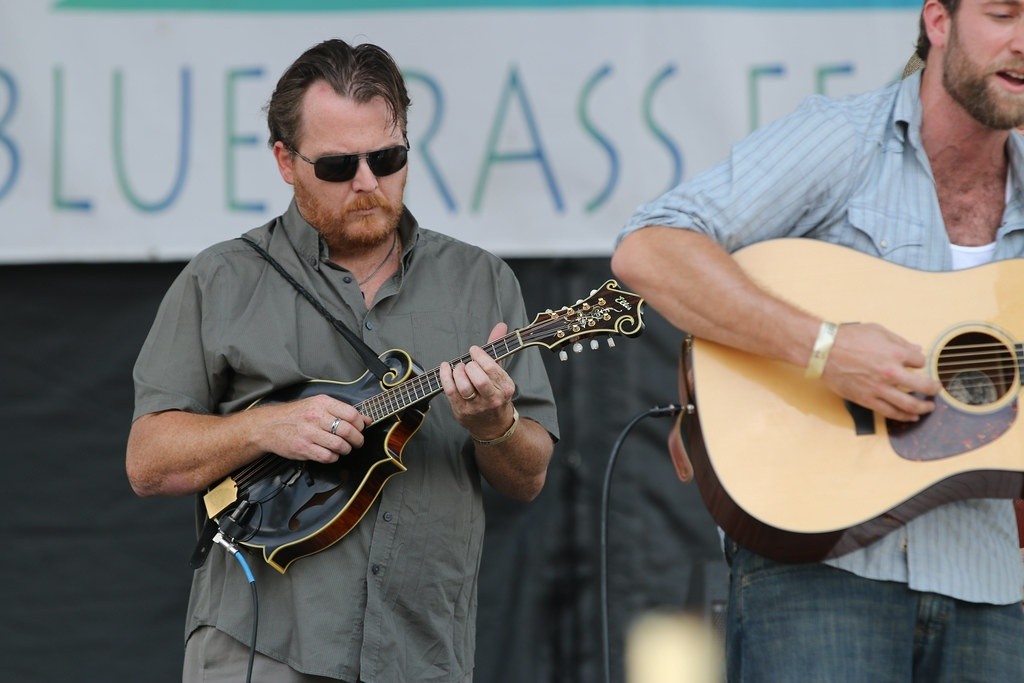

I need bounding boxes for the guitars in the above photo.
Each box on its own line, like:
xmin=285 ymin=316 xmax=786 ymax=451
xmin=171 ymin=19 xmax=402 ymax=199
xmin=677 ymin=234 xmax=1024 ymax=563
xmin=203 ymin=277 xmax=647 ymax=579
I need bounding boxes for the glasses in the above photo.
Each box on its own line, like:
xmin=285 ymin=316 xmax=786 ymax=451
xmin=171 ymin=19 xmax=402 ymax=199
xmin=285 ymin=134 xmax=411 ymax=182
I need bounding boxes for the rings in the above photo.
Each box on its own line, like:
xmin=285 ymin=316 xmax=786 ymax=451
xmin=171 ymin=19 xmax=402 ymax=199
xmin=461 ymin=393 xmax=477 ymax=401
xmin=330 ymin=415 xmax=341 ymax=435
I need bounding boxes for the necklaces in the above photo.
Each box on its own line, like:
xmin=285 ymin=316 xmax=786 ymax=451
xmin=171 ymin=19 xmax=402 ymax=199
xmin=358 ymin=232 xmax=397 ymax=300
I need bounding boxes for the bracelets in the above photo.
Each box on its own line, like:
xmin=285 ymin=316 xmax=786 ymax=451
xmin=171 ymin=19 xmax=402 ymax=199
xmin=801 ymin=318 xmax=837 ymax=386
xmin=468 ymin=408 xmax=519 ymax=446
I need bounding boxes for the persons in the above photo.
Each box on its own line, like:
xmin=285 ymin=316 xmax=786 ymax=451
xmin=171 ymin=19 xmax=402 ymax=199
xmin=611 ymin=2 xmax=1024 ymax=683
xmin=126 ymin=37 xmax=555 ymax=682
